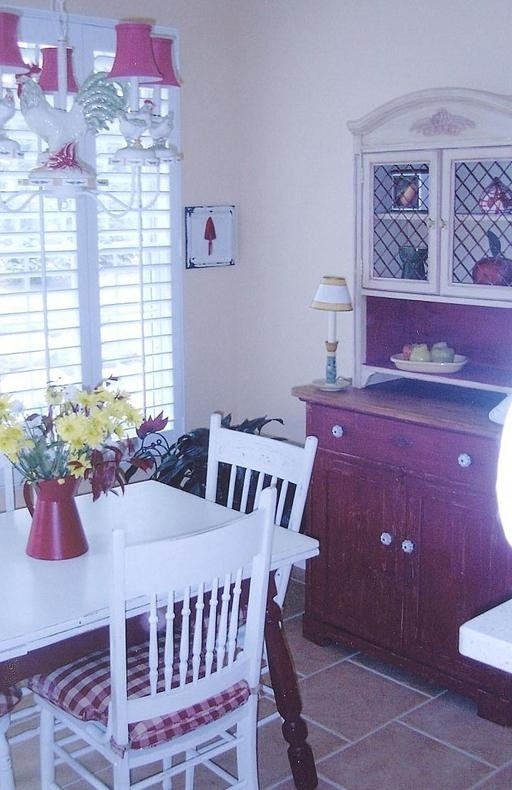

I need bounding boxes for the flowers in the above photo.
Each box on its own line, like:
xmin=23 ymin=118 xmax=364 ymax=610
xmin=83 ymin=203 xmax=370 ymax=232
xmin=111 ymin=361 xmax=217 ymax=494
xmin=0 ymin=375 xmax=169 ymax=502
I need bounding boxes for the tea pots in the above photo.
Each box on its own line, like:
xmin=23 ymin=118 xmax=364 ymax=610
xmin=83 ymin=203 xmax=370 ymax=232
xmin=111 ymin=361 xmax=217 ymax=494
xmin=22 ymin=475 xmax=91 ymax=561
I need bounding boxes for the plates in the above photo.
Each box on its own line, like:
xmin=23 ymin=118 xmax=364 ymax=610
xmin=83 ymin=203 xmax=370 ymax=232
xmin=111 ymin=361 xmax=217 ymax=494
xmin=390 ymin=352 xmax=469 ymax=375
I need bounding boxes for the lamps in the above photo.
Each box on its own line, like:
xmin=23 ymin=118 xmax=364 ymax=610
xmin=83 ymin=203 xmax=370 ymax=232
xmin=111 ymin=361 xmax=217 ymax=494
xmin=310 ymin=275 xmax=354 ymax=394
xmin=1 ymin=0 xmax=183 ymax=217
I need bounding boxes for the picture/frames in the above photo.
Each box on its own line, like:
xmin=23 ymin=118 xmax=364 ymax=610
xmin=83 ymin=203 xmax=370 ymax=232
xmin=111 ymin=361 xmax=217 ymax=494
xmin=184 ymin=204 xmax=237 ymax=270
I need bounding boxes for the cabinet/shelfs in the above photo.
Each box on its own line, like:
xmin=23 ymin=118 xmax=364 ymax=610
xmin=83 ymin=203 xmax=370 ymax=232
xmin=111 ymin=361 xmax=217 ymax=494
xmin=345 ymin=85 xmax=512 ymax=431
xmin=302 ymin=404 xmax=511 ymax=729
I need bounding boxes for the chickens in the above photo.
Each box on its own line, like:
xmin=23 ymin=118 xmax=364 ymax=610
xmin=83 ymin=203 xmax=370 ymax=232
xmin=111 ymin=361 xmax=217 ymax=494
xmin=130 ymin=110 xmax=174 ymax=149
xmin=0 ymin=90 xmax=18 ymax=135
xmin=11 ymin=60 xmax=128 ymax=173
xmin=115 ymin=101 xmax=155 ymax=148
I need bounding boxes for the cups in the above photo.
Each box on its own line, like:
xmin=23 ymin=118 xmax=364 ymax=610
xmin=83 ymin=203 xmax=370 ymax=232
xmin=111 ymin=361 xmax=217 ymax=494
xmin=398 ymin=244 xmax=428 ymax=280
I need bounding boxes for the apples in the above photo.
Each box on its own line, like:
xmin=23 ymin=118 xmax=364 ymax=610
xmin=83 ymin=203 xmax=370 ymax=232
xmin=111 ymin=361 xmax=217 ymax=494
xmin=403 ymin=339 xmax=456 ymax=365
xmin=472 ymin=257 xmax=512 ymax=285
